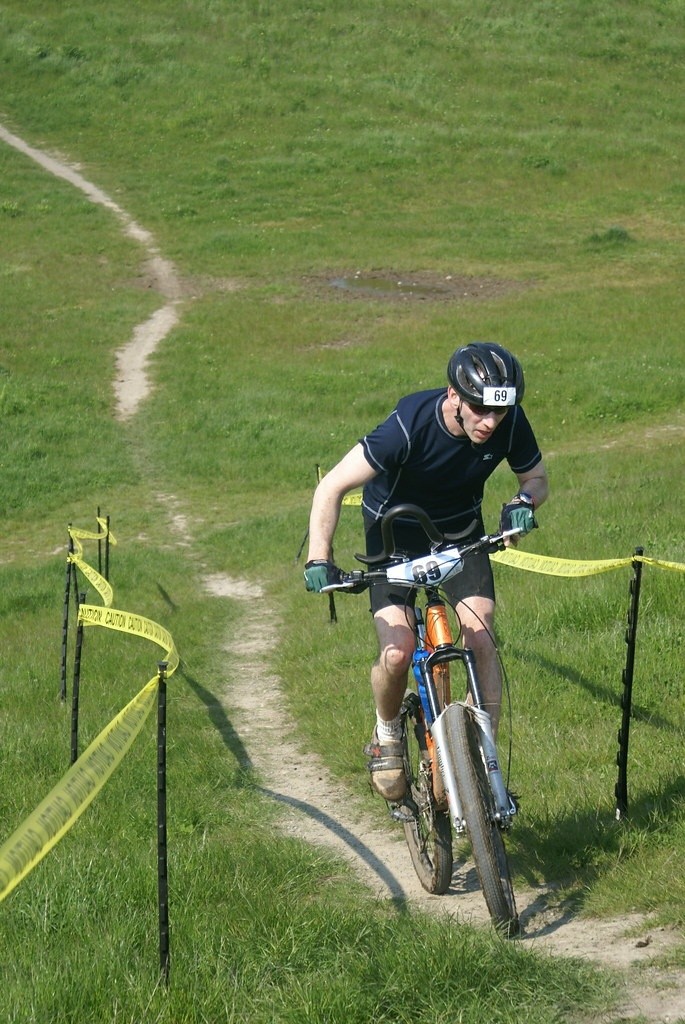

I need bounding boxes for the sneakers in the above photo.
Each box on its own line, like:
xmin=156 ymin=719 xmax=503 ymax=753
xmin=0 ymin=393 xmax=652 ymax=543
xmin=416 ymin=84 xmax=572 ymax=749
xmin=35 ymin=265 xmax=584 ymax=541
xmin=371 ymin=723 xmax=409 ymax=800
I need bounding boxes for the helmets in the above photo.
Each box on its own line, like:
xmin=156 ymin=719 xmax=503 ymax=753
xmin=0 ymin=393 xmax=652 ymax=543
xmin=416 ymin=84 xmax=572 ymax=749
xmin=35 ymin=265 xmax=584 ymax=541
xmin=446 ymin=341 xmax=525 ymax=405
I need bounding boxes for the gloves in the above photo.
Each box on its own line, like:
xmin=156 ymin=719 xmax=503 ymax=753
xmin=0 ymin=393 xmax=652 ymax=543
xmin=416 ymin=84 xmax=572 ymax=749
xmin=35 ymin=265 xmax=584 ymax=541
xmin=502 ymin=504 xmax=539 ymax=546
xmin=304 ymin=560 xmax=331 ymax=593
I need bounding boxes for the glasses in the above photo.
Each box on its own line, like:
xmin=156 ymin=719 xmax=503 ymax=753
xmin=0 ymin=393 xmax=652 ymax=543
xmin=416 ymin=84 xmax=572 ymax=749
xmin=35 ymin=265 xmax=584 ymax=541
xmin=468 ymin=404 xmax=508 ymax=415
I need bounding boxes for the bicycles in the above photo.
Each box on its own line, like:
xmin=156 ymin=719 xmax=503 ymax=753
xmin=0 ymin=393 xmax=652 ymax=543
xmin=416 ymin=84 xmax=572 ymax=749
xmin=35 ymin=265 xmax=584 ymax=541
xmin=318 ymin=527 xmax=521 ymax=942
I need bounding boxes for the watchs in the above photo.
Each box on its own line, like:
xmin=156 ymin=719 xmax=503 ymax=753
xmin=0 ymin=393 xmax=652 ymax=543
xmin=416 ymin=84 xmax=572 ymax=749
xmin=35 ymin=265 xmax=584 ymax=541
xmin=519 ymin=492 xmax=535 ymax=505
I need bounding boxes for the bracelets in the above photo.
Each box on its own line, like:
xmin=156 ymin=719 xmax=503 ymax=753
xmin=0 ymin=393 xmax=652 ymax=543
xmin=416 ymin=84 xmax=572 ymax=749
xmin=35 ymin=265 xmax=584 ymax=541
xmin=302 ymin=341 xmax=550 ymax=817
